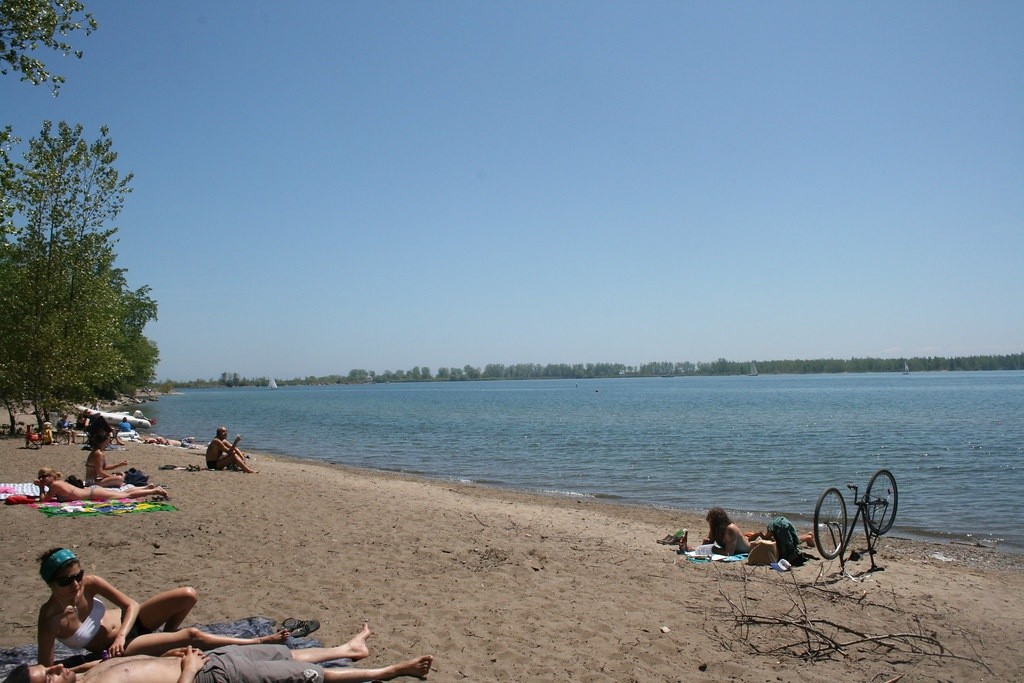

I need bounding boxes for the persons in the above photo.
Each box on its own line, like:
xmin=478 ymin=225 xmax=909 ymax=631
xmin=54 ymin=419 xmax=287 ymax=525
xmin=206 ymin=426 xmax=260 ymax=474
xmin=9 ymin=624 xmax=433 ymax=683
xmin=34 ymin=468 xmax=168 ymax=502
xmin=90 ymin=398 xmax=100 ymax=410
xmin=702 ymin=507 xmax=816 ymax=556
xmin=86 ymin=430 xmax=128 ymax=488
xmin=36 ymin=547 xmax=290 ymax=668
xmin=119 ymin=417 xmax=131 ymax=432
xmin=42 ymin=408 xmax=125 ymax=445
xmin=143 ymin=436 xmax=205 ymax=450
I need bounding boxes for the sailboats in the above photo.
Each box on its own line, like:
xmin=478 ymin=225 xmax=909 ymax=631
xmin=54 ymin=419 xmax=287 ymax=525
xmin=749 ymin=364 xmax=759 ymax=376
xmin=265 ymin=377 xmax=279 ymax=390
xmin=902 ymin=362 xmax=911 ymax=375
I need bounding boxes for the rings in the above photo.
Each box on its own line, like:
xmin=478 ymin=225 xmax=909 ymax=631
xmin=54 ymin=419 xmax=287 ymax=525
xmin=115 ymin=650 xmax=120 ymax=653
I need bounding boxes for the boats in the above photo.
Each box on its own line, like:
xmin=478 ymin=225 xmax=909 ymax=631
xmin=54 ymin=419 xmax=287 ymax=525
xmin=74 ymin=405 xmax=152 ymax=429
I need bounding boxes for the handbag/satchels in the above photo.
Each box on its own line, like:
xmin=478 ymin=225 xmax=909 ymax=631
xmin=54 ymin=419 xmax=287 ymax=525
xmin=679 ymin=530 xmax=695 ymax=552
xmin=124 ymin=468 xmax=149 ymax=487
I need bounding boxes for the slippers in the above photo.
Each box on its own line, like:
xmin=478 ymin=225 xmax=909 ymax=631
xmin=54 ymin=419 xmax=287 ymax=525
xmin=291 ymin=620 xmax=320 ymax=638
xmin=277 ymin=618 xmax=301 ymax=634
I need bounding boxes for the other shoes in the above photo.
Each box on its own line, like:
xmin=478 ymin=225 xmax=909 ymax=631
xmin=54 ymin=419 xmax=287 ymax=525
xmin=186 ymin=464 xmax=200 ymax=472
xmin=656 ymin=534 xmax=683 ymax=545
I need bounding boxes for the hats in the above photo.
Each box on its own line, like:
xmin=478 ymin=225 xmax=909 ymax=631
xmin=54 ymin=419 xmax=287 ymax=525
xmin=770 ymin=559 xmax=791 ymax=572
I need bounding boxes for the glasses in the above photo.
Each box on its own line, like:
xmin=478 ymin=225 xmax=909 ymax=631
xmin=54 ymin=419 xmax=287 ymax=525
xmin=38 ymin=475 xmax=51 ymax=479
xmin=50 ymin=569 xmax=84 ymax=587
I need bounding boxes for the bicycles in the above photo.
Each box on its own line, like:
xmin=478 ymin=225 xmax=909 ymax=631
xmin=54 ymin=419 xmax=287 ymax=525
xmin=810 ymin=469 xmax=899 ymax=586
